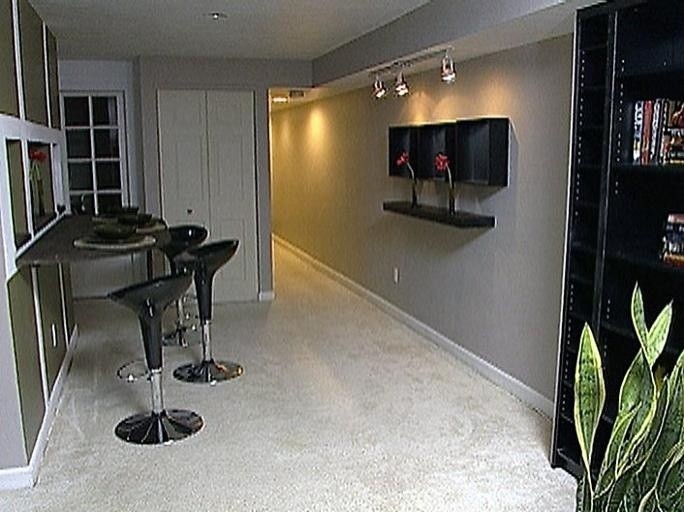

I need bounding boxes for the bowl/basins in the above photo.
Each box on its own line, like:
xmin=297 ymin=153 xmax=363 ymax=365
xmin=93 ymin=224 xmax=137 ymax=240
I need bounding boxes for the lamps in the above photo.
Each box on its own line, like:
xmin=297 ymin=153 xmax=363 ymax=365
xmin=371 ymin=51 xmax=455 ymax=100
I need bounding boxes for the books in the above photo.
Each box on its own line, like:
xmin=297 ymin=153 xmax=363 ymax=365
xmin=661 ymin=214 xmax=684 ymax=266
xmin=631 ymin=100 xmax=683 ymax=166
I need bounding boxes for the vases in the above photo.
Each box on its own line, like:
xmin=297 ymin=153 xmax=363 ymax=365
xmin=39 ymin=180 xmax=44 ymax=217
xmin=406 ymin=184 xmax=419 ymax=208
xmin=30 ymin=177 xmax=36 ymax=224
xmin=446 ymin=189 xmax=457 ymax=216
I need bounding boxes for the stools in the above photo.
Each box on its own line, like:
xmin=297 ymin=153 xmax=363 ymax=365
xmin=157 ymin=225 xmax=205 ymax=345
xmin=107 ymin=271 xmax=202 ymax=443
xmin=174 ymin=240 xmax=243 ymax=384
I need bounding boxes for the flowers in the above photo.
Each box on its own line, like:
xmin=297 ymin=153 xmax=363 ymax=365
xmin=434 ymin=154 xmax=456 ymax=189
xmin=396 ymin=152 xmax=414 ymax=183
xmin=30 ymin=147 xmax=45 ymax=181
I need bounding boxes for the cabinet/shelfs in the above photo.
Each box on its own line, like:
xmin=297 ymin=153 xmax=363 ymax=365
xmin=0 ymin=1 xmax=81 ymax=491
xmin=155 ymin=91 xmax=258 ymax=303
xmin=381 ymin=118 xmax=510 ymax=229
xmin=548 ymin=1 xmax=683 ymax=484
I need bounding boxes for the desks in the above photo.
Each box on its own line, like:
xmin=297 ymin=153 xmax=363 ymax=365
xmin=15 ymin=214 xmax=165 ymax=281
